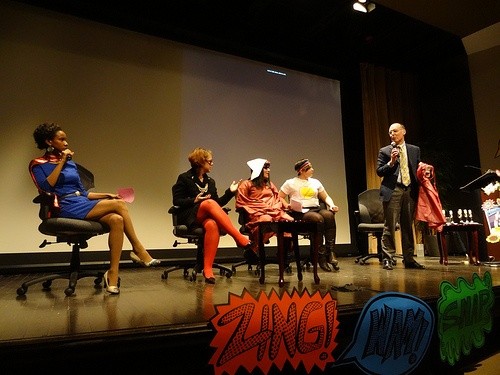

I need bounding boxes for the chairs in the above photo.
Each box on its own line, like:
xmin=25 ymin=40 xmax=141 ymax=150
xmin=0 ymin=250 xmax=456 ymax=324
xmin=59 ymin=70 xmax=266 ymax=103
xmin=231 ymin=206 xmax=293 ymax=278
xmin=288 ymin=195 xmax=340 ymax=272
xmin=354 ymin=188 xmax=403 ymax=266
xmin=161 ymin=183 xmax=234 ymax=282
xmin=16 ymin=162 xmax=121 ymax=298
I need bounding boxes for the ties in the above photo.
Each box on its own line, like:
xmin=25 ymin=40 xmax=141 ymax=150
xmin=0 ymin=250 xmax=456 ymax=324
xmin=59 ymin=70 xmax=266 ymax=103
xmin=398 ymin=145 xmax=411 ymax=187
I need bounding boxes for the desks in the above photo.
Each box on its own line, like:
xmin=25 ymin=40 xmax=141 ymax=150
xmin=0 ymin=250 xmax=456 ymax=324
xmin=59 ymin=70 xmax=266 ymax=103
xmin=436 ymin=224 xmax=483 ymax=266
xmin=257 ymin=219 xmax=325 ymax=288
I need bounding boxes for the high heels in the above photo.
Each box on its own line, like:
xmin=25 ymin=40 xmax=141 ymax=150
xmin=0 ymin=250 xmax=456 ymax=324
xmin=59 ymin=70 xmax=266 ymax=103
xmin=130 ymin=250 xmax=162 ymax=267
xmin=203 ymin=270 xmax=216 ymax=284
xmin=237 ymin=240 xmax=255 ymax=248
xmin=103 ymin=270 xmax=120 ymax=295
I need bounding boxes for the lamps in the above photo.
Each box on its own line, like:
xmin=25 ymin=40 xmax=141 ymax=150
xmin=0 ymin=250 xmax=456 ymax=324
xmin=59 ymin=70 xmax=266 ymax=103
xmin=352 ymin=0 xmax=376 ymax=13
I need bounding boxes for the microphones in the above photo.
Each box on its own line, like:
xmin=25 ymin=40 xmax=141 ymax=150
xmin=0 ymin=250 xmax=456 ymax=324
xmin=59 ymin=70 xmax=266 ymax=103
xmin=391 ymin=142 xmax=399 ymax=163
xmin=65 ymin=149 xmax=72 ymax=161
xmin=464 ymin=165 xmax=486 ymax=172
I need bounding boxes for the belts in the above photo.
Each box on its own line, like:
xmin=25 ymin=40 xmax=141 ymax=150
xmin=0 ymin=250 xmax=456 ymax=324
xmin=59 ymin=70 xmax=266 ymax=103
xmin=58 ymin=191 xmax=83 ymax=200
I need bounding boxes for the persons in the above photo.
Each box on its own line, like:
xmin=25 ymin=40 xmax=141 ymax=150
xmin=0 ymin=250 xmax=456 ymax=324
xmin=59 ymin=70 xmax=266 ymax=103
xmin=32 ymin=122 xmax=161 ymax=293
xmin=376 ymin=122 xmax=426 ymax=270
xmin=172 ymin=147 xmax=256 ymax=284
xmin=279 ymin=158 xmax=339 ymax=272
xmin=235 ymin=158 xmax=294 ymax=274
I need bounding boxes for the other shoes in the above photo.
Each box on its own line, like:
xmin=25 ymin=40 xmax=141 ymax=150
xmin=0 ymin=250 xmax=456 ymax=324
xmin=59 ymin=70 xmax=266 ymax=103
xmin=327 ymin=252 xmax=338 ymax=264
xmin=319 ymin=258 xmax=332 ymax=272
xmin=404 ymin=260 xmax=425 ymax=269
xmin=383 ymin=260 xmax=393 ymax=270
